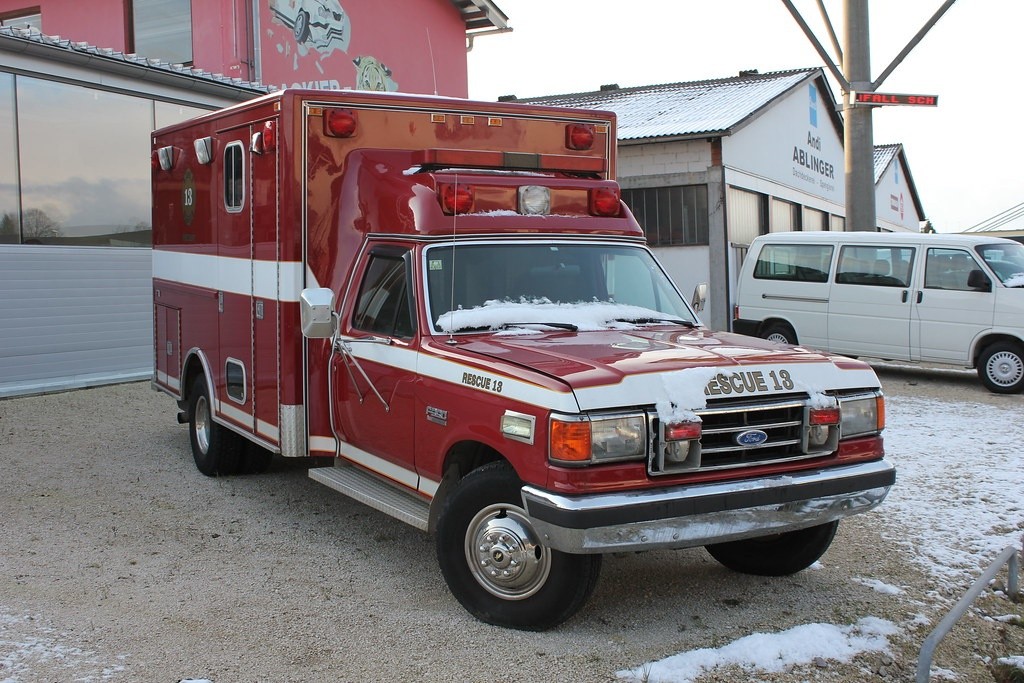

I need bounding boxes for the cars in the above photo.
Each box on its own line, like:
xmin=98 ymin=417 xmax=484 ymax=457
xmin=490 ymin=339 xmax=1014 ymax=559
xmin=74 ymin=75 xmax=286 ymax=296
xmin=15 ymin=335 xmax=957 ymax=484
xmin=268 ymin=0 xmax=347 ymax=53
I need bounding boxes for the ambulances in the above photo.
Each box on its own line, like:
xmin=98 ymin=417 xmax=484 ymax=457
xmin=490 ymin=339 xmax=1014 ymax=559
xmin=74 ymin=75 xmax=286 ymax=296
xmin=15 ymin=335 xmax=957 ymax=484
xmin=150 ymin=88 xmax=896 ymax=632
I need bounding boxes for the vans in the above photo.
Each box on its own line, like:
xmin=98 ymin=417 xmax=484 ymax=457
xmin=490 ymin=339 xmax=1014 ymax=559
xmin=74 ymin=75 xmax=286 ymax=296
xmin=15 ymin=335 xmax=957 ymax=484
xmin=733 ymin=231 xmax=1024 ymax=394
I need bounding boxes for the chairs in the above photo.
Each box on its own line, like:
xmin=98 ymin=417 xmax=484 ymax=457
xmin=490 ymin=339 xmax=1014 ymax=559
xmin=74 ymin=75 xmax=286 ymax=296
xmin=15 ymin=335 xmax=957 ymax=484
xmin=794 ymin=253 xmax=977 ymax=291
xmin=543 ymin=265 xmax=586 ymax=306
